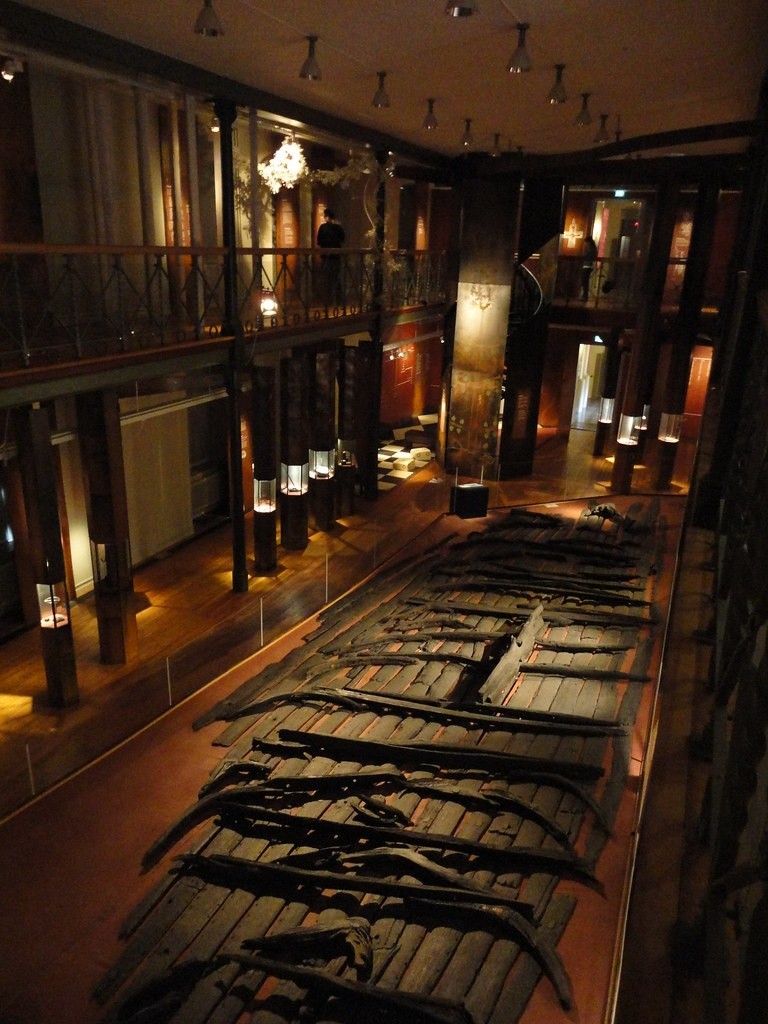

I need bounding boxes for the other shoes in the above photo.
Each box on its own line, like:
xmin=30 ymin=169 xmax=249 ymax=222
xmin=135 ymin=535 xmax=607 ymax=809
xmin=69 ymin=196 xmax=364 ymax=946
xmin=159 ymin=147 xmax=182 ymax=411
xmin=580 ymin=297 xmax=588 ymax=300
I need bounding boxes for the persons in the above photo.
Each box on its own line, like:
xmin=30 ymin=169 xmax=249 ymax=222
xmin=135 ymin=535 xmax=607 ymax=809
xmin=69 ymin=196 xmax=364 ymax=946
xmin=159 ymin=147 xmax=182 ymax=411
xmin=315 ymin=209 xmax=346 ymax=313
xmin=576 ymin=234 xmax=598 ymax=302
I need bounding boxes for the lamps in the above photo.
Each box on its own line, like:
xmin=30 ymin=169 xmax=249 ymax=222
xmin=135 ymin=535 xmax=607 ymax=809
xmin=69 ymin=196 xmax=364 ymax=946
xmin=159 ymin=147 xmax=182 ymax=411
xmin=1 ymin=61 xmax=16 ymax=81
xmin=193 ymin=0 xmax=612 ymax=158
